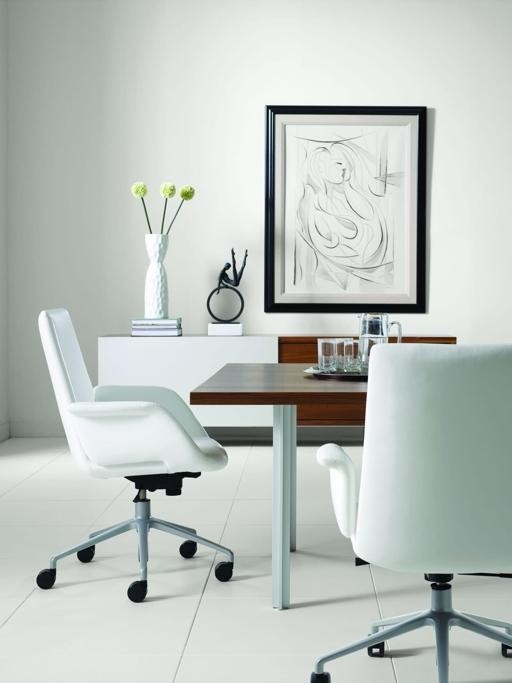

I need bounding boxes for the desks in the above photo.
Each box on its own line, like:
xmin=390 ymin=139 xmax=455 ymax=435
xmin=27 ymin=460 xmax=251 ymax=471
xmin=189 ymin=362 xmax=369 ymax=610
xmin=96 ymin=333 xmax=279 ymax=446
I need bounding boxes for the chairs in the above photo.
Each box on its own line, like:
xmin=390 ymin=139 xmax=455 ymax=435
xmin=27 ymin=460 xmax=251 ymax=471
xmin=310 ymin=342 xmax=512 ymax=683
xmin=36 ymin=305 xmax=234 ymax=602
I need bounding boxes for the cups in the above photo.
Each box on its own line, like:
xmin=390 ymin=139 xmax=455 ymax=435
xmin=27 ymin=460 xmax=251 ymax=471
xmin=318 ymin=335 xmax=354 ymax=372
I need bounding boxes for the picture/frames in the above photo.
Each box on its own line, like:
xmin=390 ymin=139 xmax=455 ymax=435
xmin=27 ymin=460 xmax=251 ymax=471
xmin=264 ymin=104 xmax=427 ymax=314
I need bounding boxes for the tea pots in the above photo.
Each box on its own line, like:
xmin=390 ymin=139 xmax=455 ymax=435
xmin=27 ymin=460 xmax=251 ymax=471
xmin=357 ymin=312 xmax=403 ymax=370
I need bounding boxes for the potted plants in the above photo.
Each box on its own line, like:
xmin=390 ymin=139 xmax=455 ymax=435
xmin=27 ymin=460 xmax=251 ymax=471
xmin=130 ymin=182 xmax=195 ymax=319
